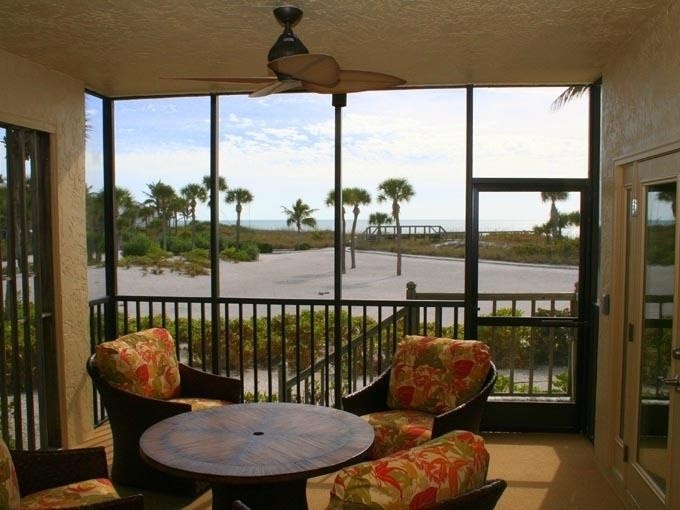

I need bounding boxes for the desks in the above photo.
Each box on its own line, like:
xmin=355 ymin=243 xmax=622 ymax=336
xmin=137 ymin=401 xmax=375 ymax=509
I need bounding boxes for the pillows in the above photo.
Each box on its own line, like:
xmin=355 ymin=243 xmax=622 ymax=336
xmin=94 ymin=327 xmax=181 ymax=401
xmin=0 ymin=438 xmax=21 ymax=509
xmin=325 ymin=429 xmax=491 ymax=510
xmin=385 ymin=333 xmax=491 ymax=413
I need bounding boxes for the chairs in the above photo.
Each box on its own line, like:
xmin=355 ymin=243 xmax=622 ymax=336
xmin=232 ymin=430 xmax=508 ymax=510
xmin=0 ymin=435 xmax=145 ymax=510
xmin=86 ymin=326 xmax=243 ymax=499
xmin=342 ymin=334 xmax=499 ymax=460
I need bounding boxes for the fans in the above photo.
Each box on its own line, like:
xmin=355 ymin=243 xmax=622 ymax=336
xmin=157 ymin=1 xmax=409 ymax=99
